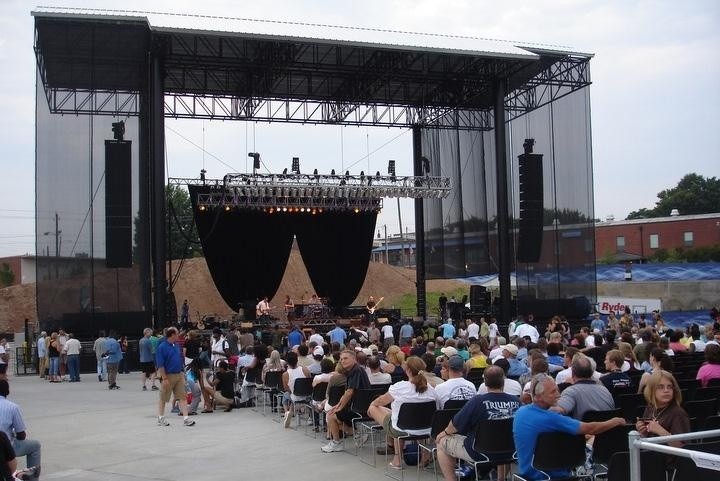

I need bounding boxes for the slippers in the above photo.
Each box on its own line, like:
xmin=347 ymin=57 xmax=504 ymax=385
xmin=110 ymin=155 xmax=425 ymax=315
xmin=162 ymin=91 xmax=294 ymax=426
xmin=387 ymin=460 xmax=403 ymax=470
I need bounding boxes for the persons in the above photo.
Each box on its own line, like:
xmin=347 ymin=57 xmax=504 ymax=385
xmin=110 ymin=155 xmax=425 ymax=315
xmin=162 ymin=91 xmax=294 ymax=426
xmin=254 ymin=296 xmax=720 ymax=480
xmin=0 ymin=336 xmax=10 ymax=380
xmin=38 ymin=327 xmax=255 ymax=427
xmin=0 ymin=380 xmax=41 ymax=481
xmin=0 ymin=431 xmax=18 ymax=481
xmin=181 ymin=300 xmax=189 ymax=323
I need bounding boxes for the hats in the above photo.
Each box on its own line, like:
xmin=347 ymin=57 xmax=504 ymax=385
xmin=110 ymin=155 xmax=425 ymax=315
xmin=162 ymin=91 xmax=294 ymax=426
xmin=440 ymin=345 xmax=458 ymax=358
xmin=312 ymin=346 xmax=325 ymax=358
xmin=500 ymin=343 xmax=519 ymax=356
xmin=447 ymin=355 xmax=465 ymax=370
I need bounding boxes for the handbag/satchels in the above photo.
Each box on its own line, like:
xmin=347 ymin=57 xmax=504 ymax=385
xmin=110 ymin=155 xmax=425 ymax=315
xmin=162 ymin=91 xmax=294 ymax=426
xmin=403 ymin=443 xmax=421 ymax=467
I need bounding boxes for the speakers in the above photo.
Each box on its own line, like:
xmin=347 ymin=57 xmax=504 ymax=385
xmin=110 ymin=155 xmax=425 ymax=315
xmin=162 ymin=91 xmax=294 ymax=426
xmin=104 ymin=140 xmax=132 ymax=268
xmin=479 ymin=292 xmax=491 ymax=312
xmin=470 ymin=285 xmax=486 ymax=313
xmin=518 ymin=154 xmax=543 ymax=263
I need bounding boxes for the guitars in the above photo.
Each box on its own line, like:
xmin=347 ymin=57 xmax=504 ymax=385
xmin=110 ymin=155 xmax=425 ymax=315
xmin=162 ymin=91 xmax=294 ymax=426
xmin=197 ymin=311 xmax=205 ymax=329
xmin=368 ymin=297 xmax=384 ymax=314
xmin=256 ymin=306 xmax=277 ymax=315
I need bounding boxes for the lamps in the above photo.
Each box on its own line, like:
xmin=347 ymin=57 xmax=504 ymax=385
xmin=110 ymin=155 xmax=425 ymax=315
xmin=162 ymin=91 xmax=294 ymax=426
xmin=195 ymin=183 xmax=452 ymax=214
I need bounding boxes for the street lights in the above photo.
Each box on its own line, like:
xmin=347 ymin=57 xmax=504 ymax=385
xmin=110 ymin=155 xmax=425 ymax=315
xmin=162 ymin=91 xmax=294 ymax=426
xmin=377 ymin=224 xmax=389 ymax=264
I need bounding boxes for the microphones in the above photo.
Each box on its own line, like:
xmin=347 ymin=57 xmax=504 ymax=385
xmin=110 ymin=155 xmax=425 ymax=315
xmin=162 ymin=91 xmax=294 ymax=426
xmin=257 ymin=298 xmax=258 ymax=300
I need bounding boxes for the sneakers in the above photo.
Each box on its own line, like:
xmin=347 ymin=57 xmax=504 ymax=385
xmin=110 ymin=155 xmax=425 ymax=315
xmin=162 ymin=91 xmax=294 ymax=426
xmin=282 ymin=411 xmax=292 ymax=428
xmin=376 ymin=444 xmax=394 ymax=455
xmin=321 ymin=439 xmax=345 ymax=453
xmin=152 ymin=385 xmax=160 ymax=392
xmin=98 ymin=374 xmax=106 ymax=381
xmin=141 ymin=385 xmax=146 ymax=391
xmin=108 ymin=383 xmax=120 ymax=390
xmin=200 ymin=408 xmax=213 ymax=413
xmin=40 ymin=375 xmax=80 ymax=383
xmin=171 ymin=405 xmax=179 ymax=413
xmin=157 ymin=416 xmax=170 ymax=427
xmin=454 ymin=462 xmax=475 ymax=478
xmin=223 ymin=404 xmax=235 ymax=412
xmin=417 ymin=451 xmax=435 ymax=469
xmin=307 ymin=415 xmax=357 ymax=440
xmin=353 ymin=432 xmax=369 ymax=448
xmin=183 ymin=417 xmax=196 ymax=425
